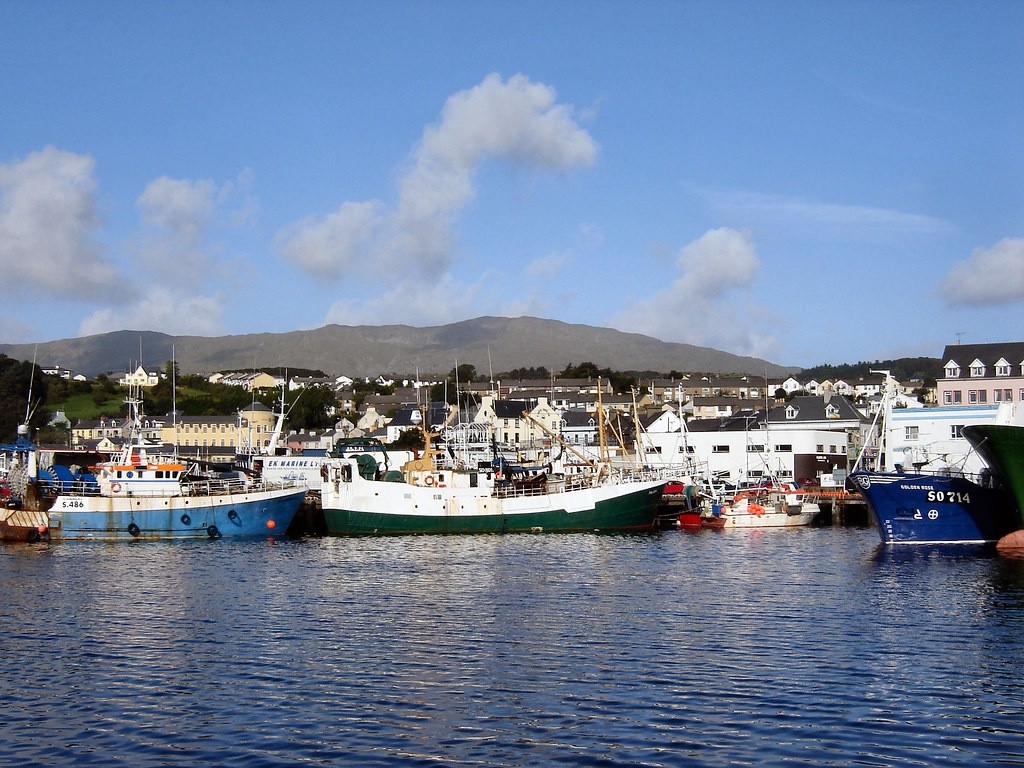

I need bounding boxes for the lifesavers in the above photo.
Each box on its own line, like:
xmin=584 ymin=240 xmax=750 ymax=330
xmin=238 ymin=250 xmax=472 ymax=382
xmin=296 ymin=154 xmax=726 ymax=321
xmin=228 ymin=510 xmax=237 ymax=519
xmin=207 ymin=525 xmax=218 ymax=537
xmin=110 ymin=481 xmax=121 ymax=493
xmin=181 ymin=514 xmax=190 ymax=523
xmin=127 ymin=523 xmax=139 ymax=534
xmin=425 ymin=475 xmax=435 ymax=485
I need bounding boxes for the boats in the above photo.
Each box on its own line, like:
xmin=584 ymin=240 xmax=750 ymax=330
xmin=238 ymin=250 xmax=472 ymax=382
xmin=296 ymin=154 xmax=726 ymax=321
xmin=842 ymin=369 xmax=1024 ymax=556
xmin=0 ymin=333 xmax=820 ymax=542
